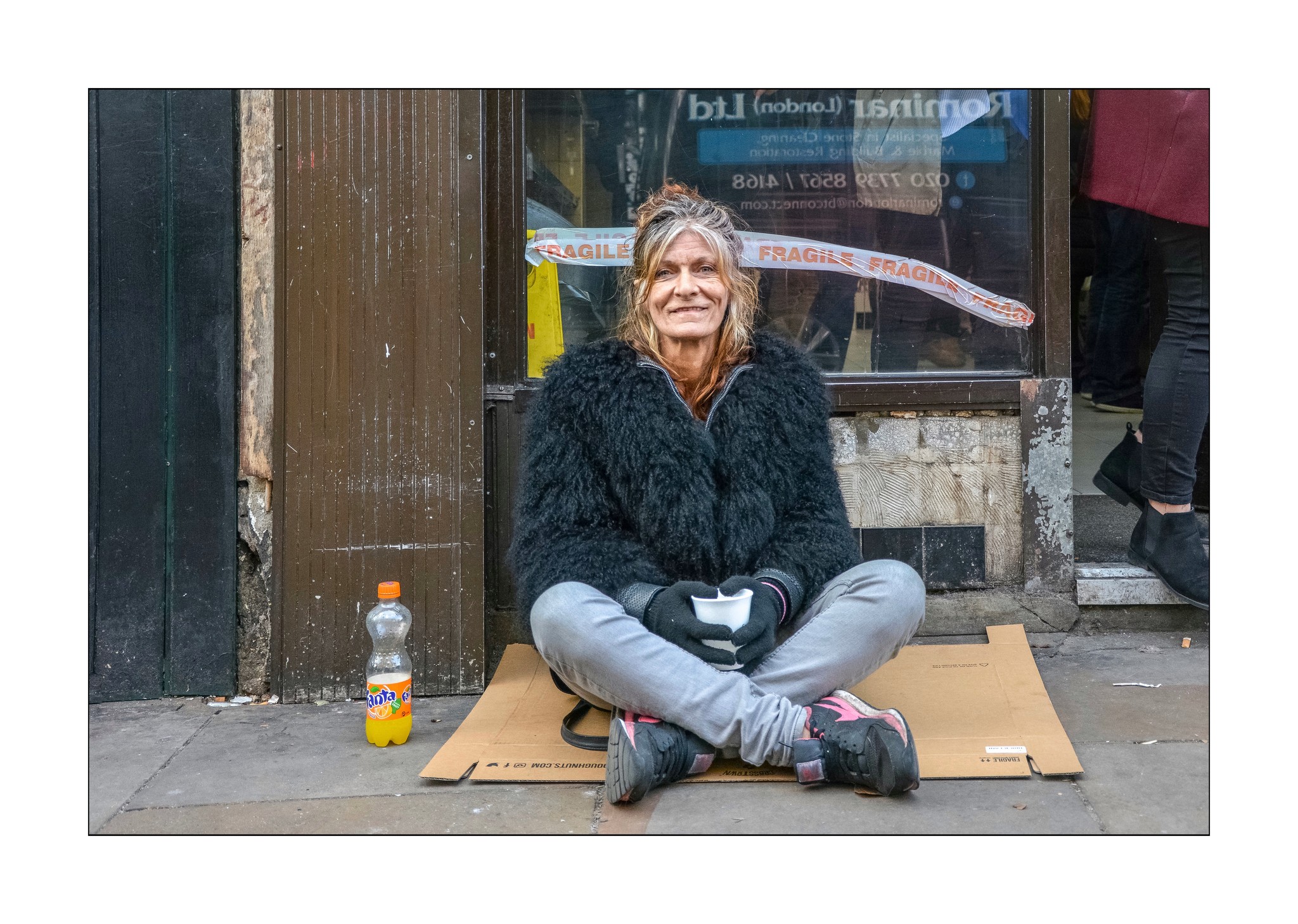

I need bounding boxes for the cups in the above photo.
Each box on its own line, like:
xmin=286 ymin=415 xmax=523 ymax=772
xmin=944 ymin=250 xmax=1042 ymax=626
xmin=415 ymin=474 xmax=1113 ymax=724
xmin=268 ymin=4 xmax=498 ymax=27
xmin=691 ymin=588 xmax=753 ymax=671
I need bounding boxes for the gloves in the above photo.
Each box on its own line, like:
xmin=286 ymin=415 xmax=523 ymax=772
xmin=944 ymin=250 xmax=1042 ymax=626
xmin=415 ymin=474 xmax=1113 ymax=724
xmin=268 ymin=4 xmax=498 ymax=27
xmin=645 ymin=580 xmax=736 ymax=666
xmin=720 ymin=576 xmax=777 ymax=676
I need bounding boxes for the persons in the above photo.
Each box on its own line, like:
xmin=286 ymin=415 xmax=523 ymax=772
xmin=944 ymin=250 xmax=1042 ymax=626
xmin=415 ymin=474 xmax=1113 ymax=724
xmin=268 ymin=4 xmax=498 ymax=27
xmin=1079 ymin=88 xmax=1210 ymax=608
xmin=854 ymin=88 xmax=1168 ymax=412
xmin=638 ymin=89 xmax=875 ymax=373
xmin=505 ymin=178 xmax=926 ymax=805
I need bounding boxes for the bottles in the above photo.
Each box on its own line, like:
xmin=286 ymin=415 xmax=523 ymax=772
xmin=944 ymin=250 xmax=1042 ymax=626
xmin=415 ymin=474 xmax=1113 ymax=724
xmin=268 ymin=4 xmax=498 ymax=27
xmin=366 ymin=581 xmax=413 ymax=747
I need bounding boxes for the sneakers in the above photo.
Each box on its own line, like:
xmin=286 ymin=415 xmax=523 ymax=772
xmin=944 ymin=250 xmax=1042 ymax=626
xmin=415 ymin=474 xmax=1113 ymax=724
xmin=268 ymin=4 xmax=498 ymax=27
xmin=606 ymin=703 xmax=719 ymax=805
xmin=792 ymin=689 xmax=922 ymax=797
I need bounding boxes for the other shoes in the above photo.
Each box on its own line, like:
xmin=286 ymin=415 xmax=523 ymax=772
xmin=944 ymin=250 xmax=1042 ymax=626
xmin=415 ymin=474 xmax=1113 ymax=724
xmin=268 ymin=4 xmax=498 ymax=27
xmin=1129 ymin=501 xmax=1210 ymax=614
xmin=1094 ymin=422 xmax=1210 ymax=513
xmin=1089 ymin=386 xmax=1146 ymax=413
xmin=1081 ymin=380 xmax=1093 ymax=399
